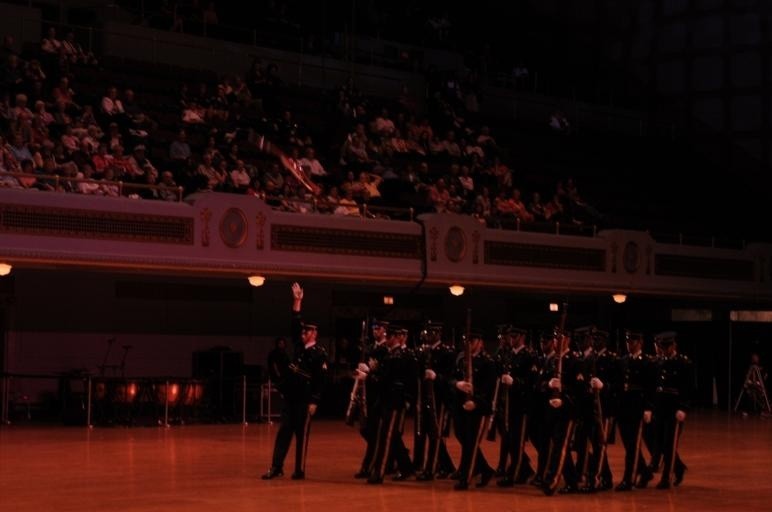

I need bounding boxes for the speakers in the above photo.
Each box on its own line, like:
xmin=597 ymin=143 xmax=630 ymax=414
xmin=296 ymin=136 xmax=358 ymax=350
xmin=191 ymin=346 xmax=242 ymax=423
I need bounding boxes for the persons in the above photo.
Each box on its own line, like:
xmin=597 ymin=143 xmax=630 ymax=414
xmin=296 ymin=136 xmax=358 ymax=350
xmin=0 ymin=27 xmax=595 ymax=232
xmin=353 ymin=319 xmax=694 ymax=490
xmin=263 ymin=283 xmax=328 ymax=479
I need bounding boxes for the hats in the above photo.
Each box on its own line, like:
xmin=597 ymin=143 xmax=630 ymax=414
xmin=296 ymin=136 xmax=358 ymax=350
xmin=461 ymin=327 xmax=484 ymax=340
xmin=625 ymin=328 xmax=643 ymax=341
xmin=423 ymin=322 xmax=443 ymax=335
xmin=386 ymin=321 xmax=408 ymax=335
xmin=589 ymin=328 xmax=610 ymax=338
xmin=299 ymin=318 xmax=320 ymax=329
xmin=654 ymin=331 xmax=677 ymax=350
xmin=507 ymin=323 xmax=530 ymax=335
xmin=539 ymin=326 xmax=591 ymax=342
xmin=370 ymin=318 xmax=391 ymax=329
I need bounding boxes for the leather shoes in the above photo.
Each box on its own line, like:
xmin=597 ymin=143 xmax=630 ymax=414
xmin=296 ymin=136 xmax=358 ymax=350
xmin=262 ymin=466 xmax=284 ymax=479
xmin=389 ymin=465 xmax=613 ymax=496
xmin=673 ymin=462 xmax=687 ymax=486
xmin=367 ymin=477 xmax=383 ymax=484
xmin=614 ymin=480 xmax=632 ymax=492
xmin=656 ymin=480 xmax=669 ymax=489
xmin=647 ymin=456 xmax=660 ymax=472
xmin=354 ymin=471 xmax=370 ymax=478
xmin=292 ymin=472 xmax=305 ymax=479
xmin=636 ymin=472 xmax=653 ymax=489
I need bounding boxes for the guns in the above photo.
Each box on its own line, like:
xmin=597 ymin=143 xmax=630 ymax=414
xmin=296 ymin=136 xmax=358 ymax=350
xmin=360 ymin=313 xmax=369 ymax=420
xmin=425 ymin=320 xmax=440 ymax=436
xmin=552 ymin=302 xmax=568 ymax=398
xmin=464 ymin=308 xmax=473 ymax=402
xmin=502 ymin=326 xmax=512 ymax=429
xmin=592 ymin=353 xmax=606 ymax=442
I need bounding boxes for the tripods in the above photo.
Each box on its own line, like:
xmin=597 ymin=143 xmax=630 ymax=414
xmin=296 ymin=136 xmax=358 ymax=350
xmin=734 ymin=364 xmax=772 ymax=415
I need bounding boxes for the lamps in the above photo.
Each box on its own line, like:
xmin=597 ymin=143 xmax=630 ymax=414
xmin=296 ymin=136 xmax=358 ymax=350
xmin=544 ymin=300 xmax=560 ymax=314
xmin=0 ymin=262 xmax=13 ymax=276
xmin=448 ymin=284 xmax=466 ymax=297
xmin=247 ymin=275 xmax=266 ymax=287
xmin=612 ymin=293 xmax=628 ymax=304
xmin=383 ymin=289 xmax=394 ymax=304
